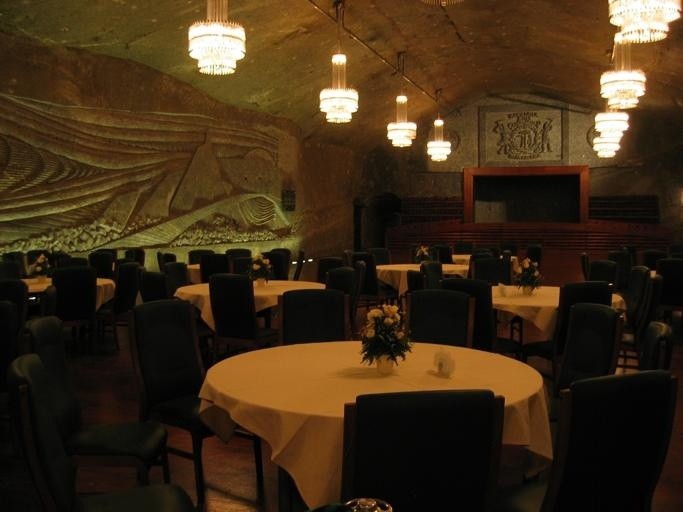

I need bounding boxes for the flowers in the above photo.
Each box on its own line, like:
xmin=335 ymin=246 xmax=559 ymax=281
xmin=357 ymin=304 xmax=413 ymax=368
xmin=246 ymin=253 xmax=272 ymax=285
xmin=414 ymin=245 xmax=431 ymax=261
xmin=511 ymin=258 xmax=544 ymax=288
xmin=26 ymin=253 xmax=53 ymax=277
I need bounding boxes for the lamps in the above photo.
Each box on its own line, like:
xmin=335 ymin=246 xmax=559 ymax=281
xmin=599 ymin=40 xmax=648 ymax=111
xmin=187 ymin=0 xmax=247 ymax=78
xmin=318 ymin=0 xmax=360 ymax=124
xmin=426 ymin=88 xmax=452 ymax=162
xmin=606 ymin=0 xmax=683 ymax=46
xmin=593 ymin=104 xmax=630 ymax=140
xmin=386 ymin=50 xmax=417 ymax=148
xmin=591 ymin=132 xmax=621 ymax=159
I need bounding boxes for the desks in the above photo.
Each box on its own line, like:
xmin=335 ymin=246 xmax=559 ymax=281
xmin=489 ymin=284 xmax=625 ymax=364
xmin=206 ymin=338 xmax=548 ymax=512
xmin=374 ymin=263 xmax=470 ymax=313
xmin=15 ymin=277 xmax=116 ymax=312
xmin=175 ymin=279 xmax=327 ymax=346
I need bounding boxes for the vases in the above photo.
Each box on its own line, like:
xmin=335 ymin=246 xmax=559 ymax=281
xmin=37 ymin=276 xmax=47 ymax=284
xmin=256 ymin=278 xmax=266 ymax=289
xmin=375 ymin=358 xmax=395 ymax=376
xmin=521 ymin=286 xmax=534 ymax=295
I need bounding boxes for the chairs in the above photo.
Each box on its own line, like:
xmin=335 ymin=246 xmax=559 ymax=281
xmin=225 ymin=248 xmax=252 ymax=260
xmin=497 ymin=239 xmax=517 ymax=258
xmin=127 ymin=298 xmax=265 ymax=512
xmin=350 ymin=251 xmax=403 ymax=311
xmin=472 ymin=247 xmax=490 ymax=254
xmin=471 ymin=256 xmax=511 ymax=288
xmin=366 ymin=247 xmax=392 ymax=266
xmin=2 ymin=251 xmax=27 ymax=279
xmin=157 ymin=251 xmax=166 ymax=272
xmin=325 ymin=266 xmax=359 ymax=319
xmin=293 ymin=251 xmax=305 ymax=282
xmin=608 ymin=250 xmax=634 ymax=287
xmin=637 ymin=320 xmax=673 ymax=371
xmin=467 ymin=251 xmax=491 ymax=278
xmin=51 ymin=265 xmax=102 ymax=364
xmin=406 ymin=270 xmax=421 ymax=294
xmin=98 ymin=262 xmax=140 ymax=351
xmin=612 ymin=265 xmax=664 ymax=369
xmin=24 ymin=314 xmax=171 ymax=488
xmin=522 ymin=279 xmax=613 ymax=381
xmin=8 ymin=354 xmax=196 ymax=512
xmin=269 ymin=248 xmax=291 ymax=281
xmin=656 ymin=257 xmax=683 ymax=286
xmin=666 ymin=243 xmax=682 ymax=257
xmin=352 ymin=260 xmax=367 ymax=319
xmin=451 ymin=254 xmax=472 ymax=264
xmin=188 ymin=250 xmax=215 ymax=266
xmin=440 ymin=275 xmax=498 ymax=352
xmin=317 ymin=256 xmax=345 ymax=284
xmin=342 ymin=249 xmax=353 ymax=268
xmin=163 ymin=253 xmax=177 ymax=265
xmin=199 ymin=254 xmax=230 ymax=284
xmin=0 ymin=276 xmax=29 ymax=347
xmin=404 ymin=288 xmax=476 ymax=350
xmin=451 ymin=240 xmax=475 ymax=255
xmin=428 ymin=242 xmax=453 ymax=264
xmin=139 ymin=271 xmax=175 ymax=304
xmin=125 ymin=247 xmax=146 ymax=267
xmin=340 ymin=387 xmax=506 ymax=512
xmin=96 ymin=248 xmax=118 ymax=263
xmin=581 ymin=251 xmax=590 ymax=280
xmin=56 ymin=257 xmax=88 ymax=269
xmin=88 ymin=251 xmax=114 ymax=279
xmin=160 ymin=262 xmax=188 ymax=282
xmin=537 ymin=367 xmax=679 ymax=512
xmin=26 ymin=250 xmax=51 ymax=277
xmin=551 ymin=301 xmax=621 ymax=401
xmin=420 ymin=261 xmax=443 ymax=291
xmin=642 ymin=248 xmax=667 ymax=271
xmin=229 ymin=256 xmax=254 ymax=278
xmin=526 ymin=245 xmax=542 ymax=268
xmin=113 ymin=258 xmax=135 ymax=277
xmin=277 ymin=288 xmax=351 ymax=347
xmin=669 ymin=251 xmax=683 ymax=258
xmin=587 ymin=259 xmax=617 ymax=283
xmin=623 ymin=245 xmax=638 ymax=267
